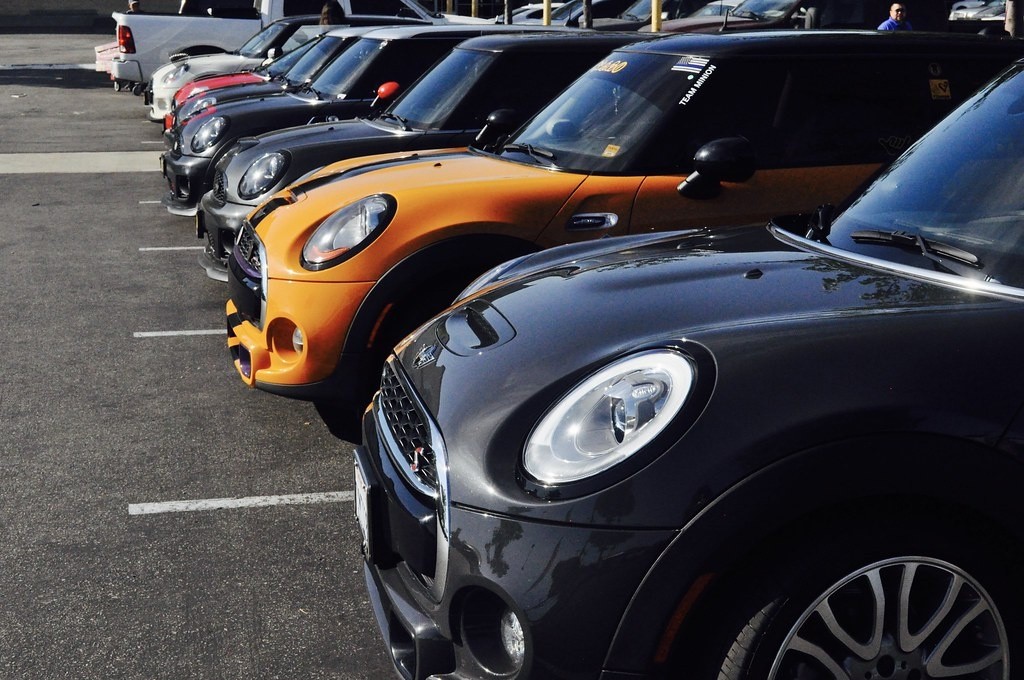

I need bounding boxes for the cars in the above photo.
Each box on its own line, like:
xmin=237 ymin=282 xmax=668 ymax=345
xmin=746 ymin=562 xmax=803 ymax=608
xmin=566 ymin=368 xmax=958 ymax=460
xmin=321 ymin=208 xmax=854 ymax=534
xmin=93 ymin=0 xmax=1024 ymax=679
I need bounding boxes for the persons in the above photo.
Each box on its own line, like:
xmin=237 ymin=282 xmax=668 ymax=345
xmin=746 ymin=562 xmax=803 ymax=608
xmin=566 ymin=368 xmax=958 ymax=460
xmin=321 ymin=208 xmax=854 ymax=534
xmin=877 ymin=4 xmax=913 ymax=32
